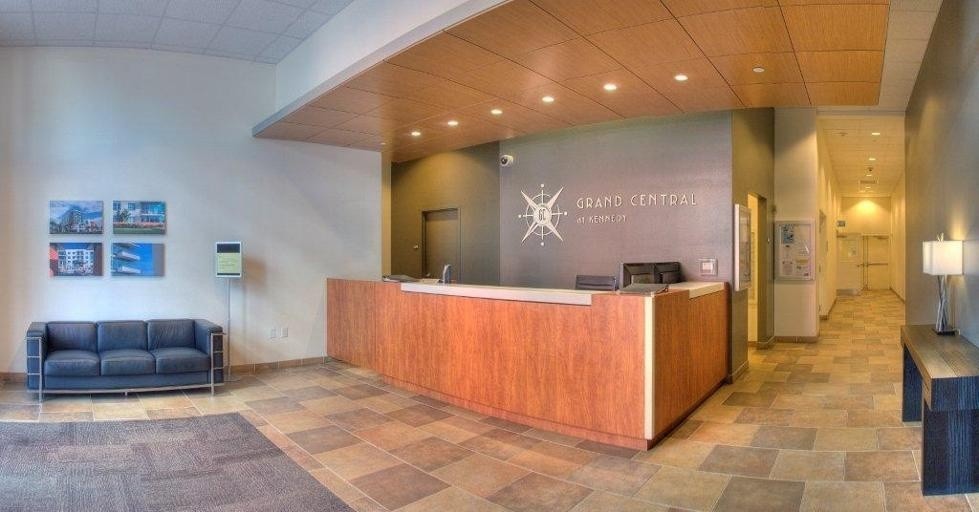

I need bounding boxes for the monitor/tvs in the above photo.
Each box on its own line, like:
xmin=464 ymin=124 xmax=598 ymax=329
xmin=654 ymin=262 xmax=681 ymax=284
xmin=443 ymin=265 xmax=451 ymax=283
xmin=619 ymin=263 xmax=654 ymax=289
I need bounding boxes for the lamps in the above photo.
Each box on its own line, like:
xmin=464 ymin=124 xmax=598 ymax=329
xmin=922 ymin=232 xmax=963 ymax=335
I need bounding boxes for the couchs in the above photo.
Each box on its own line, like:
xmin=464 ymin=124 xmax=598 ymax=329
xmin=26 ymin=318 xmax=226 ymax=401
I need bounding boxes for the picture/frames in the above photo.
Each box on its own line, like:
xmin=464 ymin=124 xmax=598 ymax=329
xmin=774 ymin=219 xmax=815 ymax=282
xmin=734 ymin=203 xmax=752 ymax=292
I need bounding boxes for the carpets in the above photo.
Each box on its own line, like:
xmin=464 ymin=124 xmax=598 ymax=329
xmin=0 ymin=412 xmax=356 ymax=512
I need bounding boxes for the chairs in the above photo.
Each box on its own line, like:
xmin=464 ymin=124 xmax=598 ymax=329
xmin=575 ymin=275 xmax=616 ymax=291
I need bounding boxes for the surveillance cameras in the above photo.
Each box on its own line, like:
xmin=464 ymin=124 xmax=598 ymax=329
xmin=500 ymin=155 xmax=513 ymax=167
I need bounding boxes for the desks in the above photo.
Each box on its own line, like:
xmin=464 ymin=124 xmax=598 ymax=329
xmin=900 ymin=325 xmax=979 ymax=496
